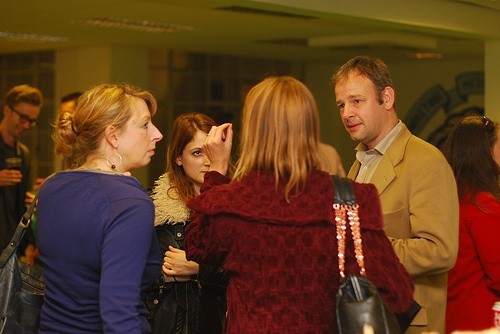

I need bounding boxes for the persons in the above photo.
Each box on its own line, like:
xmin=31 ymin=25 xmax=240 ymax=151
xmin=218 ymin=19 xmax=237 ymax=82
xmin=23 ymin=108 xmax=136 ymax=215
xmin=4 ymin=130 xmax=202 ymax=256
xmin=445 ymin=116 xmax=499 ymax=333
xmin=149 ymin=113 xmax=236 ymax=334
xmin=334 ymin=57 xmax=459 ymax=333
xmin=0 ymin=86 xmax=42 ymax=264
xmin=24 ymin=92 xmax=84 ymax=210
xmin=0 ymin=85 xmax=162 ymax=334
xmin=186 ymin=76 xmax=414 ymax=334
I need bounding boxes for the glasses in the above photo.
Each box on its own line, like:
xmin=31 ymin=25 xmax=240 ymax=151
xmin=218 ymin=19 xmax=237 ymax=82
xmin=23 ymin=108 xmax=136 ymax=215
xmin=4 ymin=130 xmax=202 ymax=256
xmin=481 ymin=115 xmax=495 ymax=131
xmin=7 ymin=104 xmax=38 ymax=128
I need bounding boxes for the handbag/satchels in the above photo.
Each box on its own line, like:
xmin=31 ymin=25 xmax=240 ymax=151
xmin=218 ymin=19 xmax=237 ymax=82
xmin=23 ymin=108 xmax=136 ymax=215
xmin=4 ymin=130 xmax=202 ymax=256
xmin=0 ymin=171 xmax=66 ymax=334
xmin=333 ymin=175 xmax=402 ymax=334
xmin=141 ymin=276 xmax=226 ymax=334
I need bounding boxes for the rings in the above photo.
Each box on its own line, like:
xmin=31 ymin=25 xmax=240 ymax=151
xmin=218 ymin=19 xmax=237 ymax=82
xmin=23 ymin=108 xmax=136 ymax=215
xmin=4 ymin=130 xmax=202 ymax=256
xmin=207 ymin=134 xmax=210 ymax=136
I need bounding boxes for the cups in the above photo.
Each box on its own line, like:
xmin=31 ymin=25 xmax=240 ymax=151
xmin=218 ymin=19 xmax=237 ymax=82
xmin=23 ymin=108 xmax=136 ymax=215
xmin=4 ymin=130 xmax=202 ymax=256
xmin=6 ymin=158 xmax=22 ymax=172
